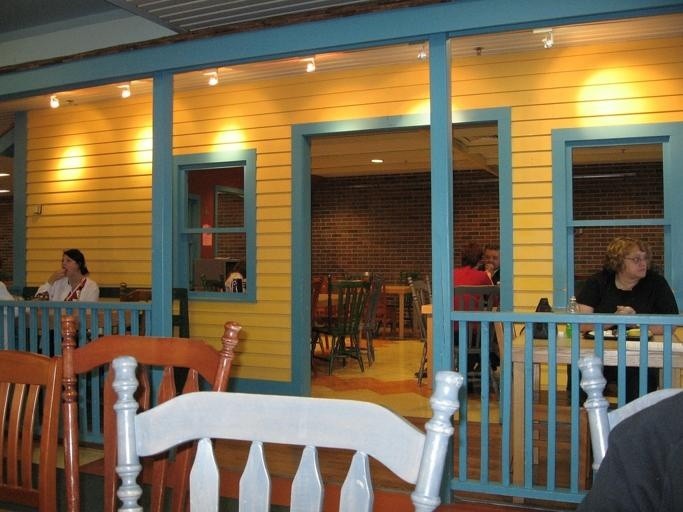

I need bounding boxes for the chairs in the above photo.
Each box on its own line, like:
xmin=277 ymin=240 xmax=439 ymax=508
xmin=110 ymin=356 xmax=462 ymax=511
xmin=491 ymin=307 xmax=683 ymax=503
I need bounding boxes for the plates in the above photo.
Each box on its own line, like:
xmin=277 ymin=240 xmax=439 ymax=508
xmin=584 ymin=327 xmax=653 ymax=341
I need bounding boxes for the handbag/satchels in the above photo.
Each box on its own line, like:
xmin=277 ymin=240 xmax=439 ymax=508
xmin=519 ymin=297 xmax=556 ymax=340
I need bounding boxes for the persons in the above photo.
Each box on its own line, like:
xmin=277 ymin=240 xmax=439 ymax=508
xmin=219 ymin=257 xmax=246 ymax=292
xmin=32 ymin=248 xmax=101 ymax=358
xmin=470 ymin=245 xmax=500 ymax=377
xmin=452 ymin=241 xmax=499 ymax=395
xmin=566 ymin=237 xmax=679 ymax=411
xmin=0 ymin=281 xmax=27 ymax=350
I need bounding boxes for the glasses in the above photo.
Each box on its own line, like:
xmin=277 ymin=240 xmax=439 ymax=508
xmin=623 ymin=255 xmax=647 ymax=264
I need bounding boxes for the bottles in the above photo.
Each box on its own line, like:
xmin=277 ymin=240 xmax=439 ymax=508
xmin=531 ymin=298 xmax=558 ymax=339
xmin=565 ymin=295 xmax=582 ymax=338
